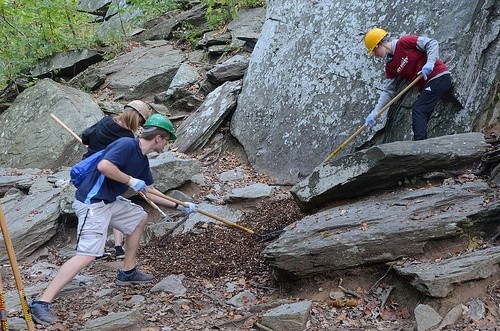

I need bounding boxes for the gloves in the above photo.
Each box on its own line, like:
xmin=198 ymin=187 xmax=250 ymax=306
xmin=127 ymin=176 xmax=147 ymax=198
xmin=422 ymin=64 xmax=434 ymax=80
xmin=365 ymin=110 xmax=383 ymax=127
xmin=175 ymin=201 xmax=199 ymax=214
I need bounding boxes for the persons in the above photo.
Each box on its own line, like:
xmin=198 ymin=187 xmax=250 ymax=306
xmin=29 ymin=113 xmax=200 ymax=326
xmin=365 ymin=28 xmax=466 ymax=141
xmin=82 ymin=100 xmax=150 ymax=260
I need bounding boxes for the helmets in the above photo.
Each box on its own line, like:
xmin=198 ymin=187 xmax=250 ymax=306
xmin=124 ymin=100 xmax=149 ymax=120
xmin=143 ymin=114 xmax=177 ymax=141
xmin=364 ymin=28 xmax=389 ymax=56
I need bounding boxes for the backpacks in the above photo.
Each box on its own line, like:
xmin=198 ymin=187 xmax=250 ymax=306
xmin=70 ymin=137 xmax=148 ymax=188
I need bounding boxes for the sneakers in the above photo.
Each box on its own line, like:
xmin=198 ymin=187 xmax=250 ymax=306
xmin=29 ymin=298 xmax=54 ymax=325
xmin=116 ymin=269 xmax=155 ymax=286
xmin=95 ymin=254 xmax=110 ymax=260
xmin=114 ymin=244 xmax=126 ymax=259
